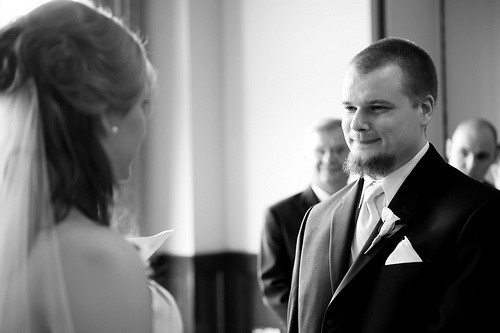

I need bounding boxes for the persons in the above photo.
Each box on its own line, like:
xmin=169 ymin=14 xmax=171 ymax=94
xmin=286 ymin=38 xmax=500 ymax=333
xmin=257 ymin=119 xmax=350 ymax=333
xmin=443 ymin=116 xmax=500 ymax=183
xmin=0 ymin=0 xmax=187 ymax=333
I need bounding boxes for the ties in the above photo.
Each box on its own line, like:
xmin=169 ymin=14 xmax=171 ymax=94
xmin=349 ymin=181 xmax=384 ymax=267
